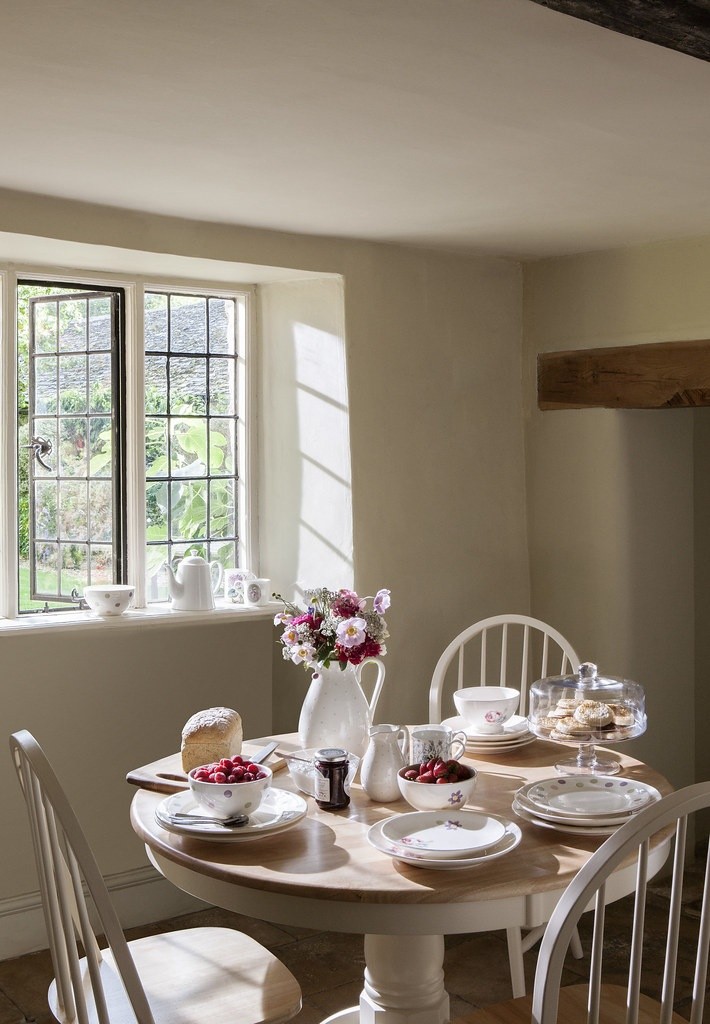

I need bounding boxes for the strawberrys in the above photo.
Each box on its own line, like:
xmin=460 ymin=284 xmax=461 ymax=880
xmin=402 ymin=756 xmax=471 ymax=784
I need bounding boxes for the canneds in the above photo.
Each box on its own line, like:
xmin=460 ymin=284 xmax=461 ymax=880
xmin=315 ymin=749 xmax=351 ymax=810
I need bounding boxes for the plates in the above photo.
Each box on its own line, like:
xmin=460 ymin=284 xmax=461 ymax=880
xmin=153 ymin=788 xmax=308 ymax=843
xmin=441 ymin=713 xmax=538 ymax=754
xmin=366 ymin=808 xmax=522 ymax=871
xmin=512 ymin=775 xmax=663 ymax=835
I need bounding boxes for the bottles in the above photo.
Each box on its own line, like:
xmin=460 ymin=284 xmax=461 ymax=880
xmin=313 ymin=748 xmax=351 ymax=810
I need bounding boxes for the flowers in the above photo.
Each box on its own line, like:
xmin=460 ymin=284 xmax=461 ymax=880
xmin=272 ymin=587 xmax=392 ymax=679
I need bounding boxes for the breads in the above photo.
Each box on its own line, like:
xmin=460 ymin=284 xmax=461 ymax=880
xmin=181 ymin=707 xmax=243 ymax=772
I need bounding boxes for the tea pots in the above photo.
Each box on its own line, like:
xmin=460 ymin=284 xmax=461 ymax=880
xmin=165 ymin=549 xmax=223 ymax=612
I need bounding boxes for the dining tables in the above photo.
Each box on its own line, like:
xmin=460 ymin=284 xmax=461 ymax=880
xmin=132 ymin=725 xmax=679 ymax=1024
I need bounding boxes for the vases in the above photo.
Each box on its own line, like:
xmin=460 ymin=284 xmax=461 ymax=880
xmin=297 ymin=656 xmax=386 ymax=759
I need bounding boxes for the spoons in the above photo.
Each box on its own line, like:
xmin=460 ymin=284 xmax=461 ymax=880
xmin=168 ymin=812 xmax=249 ymax=827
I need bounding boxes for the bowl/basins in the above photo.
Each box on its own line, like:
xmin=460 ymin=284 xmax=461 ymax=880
xmin=285 ymin=747 xmax=360 ymax=795
xmin=396 ymin=764 xmax=477 ymax=812
xmin=453 ymin=686 xmax=520 ymax=734
xmin=187 ymin=759 xmax=273 ymax=816
xmin=406 ymin=724 xmax=467 ymax=763
xmin=83 ymin=584 xmax=136 ymax=615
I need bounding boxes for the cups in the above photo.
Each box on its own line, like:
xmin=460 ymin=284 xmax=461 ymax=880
xmin=234 ymin=578 xmax=270 ymax=606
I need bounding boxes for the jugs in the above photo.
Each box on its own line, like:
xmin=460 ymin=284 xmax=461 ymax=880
xmin=359 ymin=724 xmax=410 ymax=803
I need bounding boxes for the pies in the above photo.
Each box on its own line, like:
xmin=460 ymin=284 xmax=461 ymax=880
xmin=532 ymin=698 xmax=635 ymax=743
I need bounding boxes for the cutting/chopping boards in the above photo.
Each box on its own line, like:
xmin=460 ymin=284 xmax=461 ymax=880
xmin=125 ymin=740 xmax=288 ymax=795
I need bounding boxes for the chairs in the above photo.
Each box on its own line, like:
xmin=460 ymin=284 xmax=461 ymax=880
xmin=446 ymin=782 xmax=710 ymax=1024
xmin=429 ymin=614 xmax=584 ymax=999
xmin=8 ymin=730 xmax=303 ymax=1024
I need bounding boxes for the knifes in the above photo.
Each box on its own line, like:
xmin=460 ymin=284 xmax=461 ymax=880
xmin=251 ymin=742 xmax=280 ymax=765
